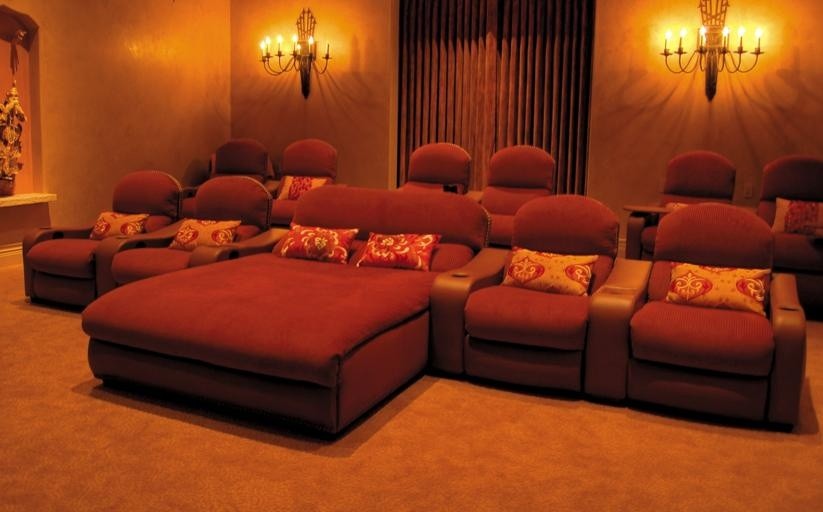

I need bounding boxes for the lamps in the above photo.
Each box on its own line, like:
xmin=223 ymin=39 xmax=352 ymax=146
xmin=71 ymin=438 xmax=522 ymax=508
xmin=661 ymin=0 xmax=763 ymax=102
xmin=260 ymin=8 xmax=331 ymax=99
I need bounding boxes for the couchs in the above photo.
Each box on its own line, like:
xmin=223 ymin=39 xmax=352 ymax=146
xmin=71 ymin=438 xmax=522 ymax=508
xmin=400 ymin=142 xmax=823 ymax=435
xmin=23 ymin=137 xmax=339 ymax=313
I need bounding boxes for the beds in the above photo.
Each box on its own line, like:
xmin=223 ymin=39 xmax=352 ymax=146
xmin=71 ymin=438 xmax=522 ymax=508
xmin=82 ymin=182 xmax=492 ymax=434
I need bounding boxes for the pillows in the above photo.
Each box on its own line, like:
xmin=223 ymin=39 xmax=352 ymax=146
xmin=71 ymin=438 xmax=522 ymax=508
xmin=273 ymin=221 xmax=359 ymax=265
xmin=355 ymin=230 xmax=443 ymax=272
